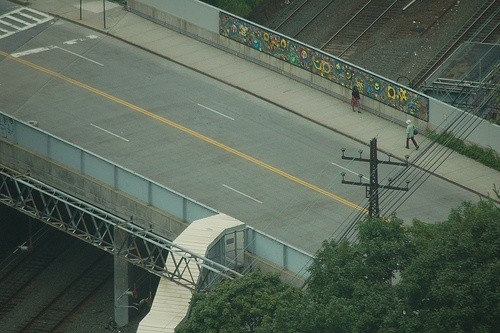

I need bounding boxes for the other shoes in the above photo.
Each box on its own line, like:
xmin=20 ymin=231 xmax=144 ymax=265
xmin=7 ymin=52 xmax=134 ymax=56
xmin=353 ymin=110 xmax=355 ymax=112
xmin=358 ymin=111 xmax=361 ymax=112
xmin=416 ymin=146 xmax=419 ymax=150
xmin=405 ymin=146 xmax=409 ymax=149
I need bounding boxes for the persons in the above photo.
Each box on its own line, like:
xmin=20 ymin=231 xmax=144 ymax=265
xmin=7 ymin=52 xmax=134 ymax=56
xmin=405 ymin=118 xmax=419 ymax=150
xmin=351 ymin=84 xmax=362 ymax=112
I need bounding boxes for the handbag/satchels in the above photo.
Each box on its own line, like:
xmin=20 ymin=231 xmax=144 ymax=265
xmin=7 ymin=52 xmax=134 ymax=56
xmin=413 ymin=126 xmax=417 ymax=135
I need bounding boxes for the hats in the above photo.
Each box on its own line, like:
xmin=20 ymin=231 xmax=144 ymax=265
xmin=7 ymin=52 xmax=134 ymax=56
xmin=406 ymin=120 xmax=411 ymax=125
